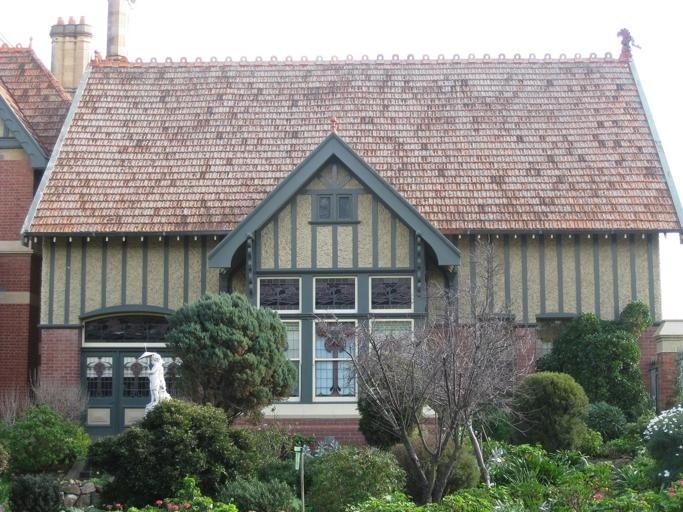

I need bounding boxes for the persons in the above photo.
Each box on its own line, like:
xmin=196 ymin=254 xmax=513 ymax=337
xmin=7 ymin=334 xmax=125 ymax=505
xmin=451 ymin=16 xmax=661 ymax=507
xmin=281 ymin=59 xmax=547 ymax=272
xmin=142 ymin=354 xmax=166 ymax=405
